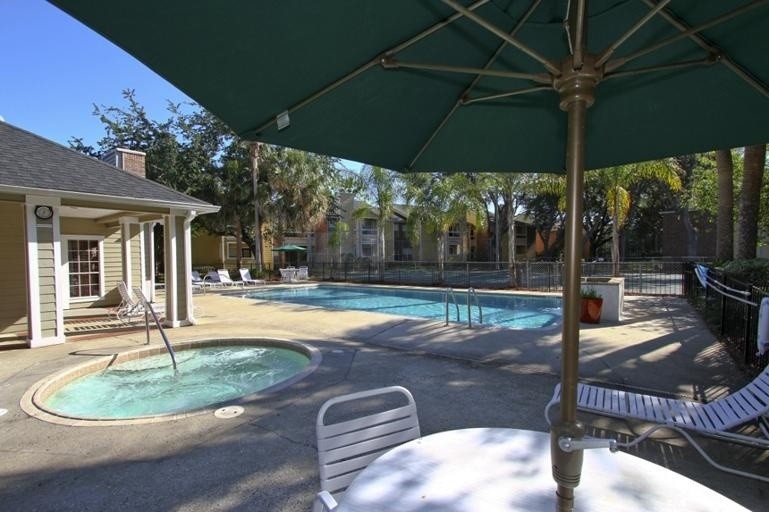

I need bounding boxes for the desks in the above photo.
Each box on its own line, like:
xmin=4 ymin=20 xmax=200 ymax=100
xmin=336 ymin=427 xmax=752 ymax=512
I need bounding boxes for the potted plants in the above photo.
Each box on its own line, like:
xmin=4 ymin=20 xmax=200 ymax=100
xmin=580 ymin=287 xmax=603 ymax=324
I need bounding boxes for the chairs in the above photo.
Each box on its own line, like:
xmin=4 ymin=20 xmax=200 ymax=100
xmin=544 ymin=366 xmax=769 ymax=482
xmin=279 ymin=265 xmax=308 ymax=285
xmin=191 ymin=267 xmax=265 ymax=294
xmin=312 ymin=386 xmax=421 ymax=512
xmin=120 ymin=285 xmax=204 ymax=326
xmin=108 ymin=282 xmax=166 ymax=322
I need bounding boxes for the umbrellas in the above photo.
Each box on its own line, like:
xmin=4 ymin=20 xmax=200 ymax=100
xmin=271 ymin=243 xmax=308 ymax=267
